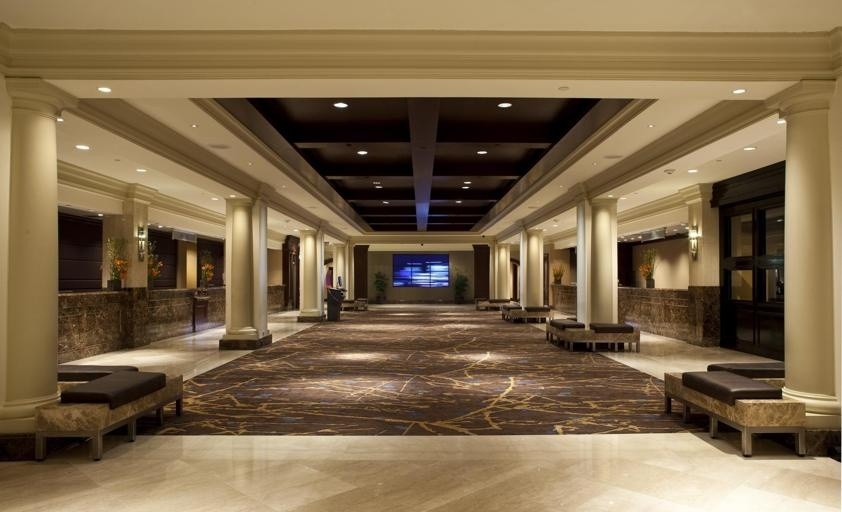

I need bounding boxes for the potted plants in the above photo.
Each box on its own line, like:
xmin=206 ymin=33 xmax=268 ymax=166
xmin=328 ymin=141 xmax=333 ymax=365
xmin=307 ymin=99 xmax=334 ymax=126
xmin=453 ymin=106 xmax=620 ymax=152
xmin=374 ymin=270 xmax=388 ymax=304
xmin=552 ymin=262 xmax=567 ymax=285
xmin=640 ymin=247 xmax=657 ymax=289
xmin=197 ymin=250 xmax=216 ymax=282
xmin=452 ymin=268 xmax=470 ymax=302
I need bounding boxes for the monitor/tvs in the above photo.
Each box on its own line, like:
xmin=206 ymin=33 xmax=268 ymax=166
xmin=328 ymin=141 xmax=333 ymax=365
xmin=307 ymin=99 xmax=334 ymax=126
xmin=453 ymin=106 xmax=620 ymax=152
xmin=338 ymin=276 xmax=342 ymax=286
xmin=392 ymin=253 xmax=449 ymax=288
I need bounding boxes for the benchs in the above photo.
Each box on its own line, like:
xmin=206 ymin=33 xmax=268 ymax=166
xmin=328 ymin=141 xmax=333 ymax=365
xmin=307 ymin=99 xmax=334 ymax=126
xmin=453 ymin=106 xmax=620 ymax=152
xmin=35 ymin=363 xmax=183 ymax=461
xmin=664 ymin=360 xmax=807 ymax=456
xmin=545 ymin=316 xmax=641 ymax=353
xmin=500 ymin=303 xmax=553 ymax=324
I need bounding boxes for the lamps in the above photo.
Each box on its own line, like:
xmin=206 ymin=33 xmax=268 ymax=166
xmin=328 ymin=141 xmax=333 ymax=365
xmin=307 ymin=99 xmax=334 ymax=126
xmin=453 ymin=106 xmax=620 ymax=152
xmin=688 ymin=226 xmax=699 ymax=260
xmin=138 ymin=227 xmax=147 ymax=263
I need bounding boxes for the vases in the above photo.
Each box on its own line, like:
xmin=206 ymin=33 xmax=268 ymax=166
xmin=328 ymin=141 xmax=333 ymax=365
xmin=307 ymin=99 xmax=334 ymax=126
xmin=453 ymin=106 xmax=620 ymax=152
xmin=200 ymin=279 xmax=206 ymax=288
xmin=147 ymin=278 xmax=154 ymax=290
xmin=107 ymin=278 xmax=122 ymax=291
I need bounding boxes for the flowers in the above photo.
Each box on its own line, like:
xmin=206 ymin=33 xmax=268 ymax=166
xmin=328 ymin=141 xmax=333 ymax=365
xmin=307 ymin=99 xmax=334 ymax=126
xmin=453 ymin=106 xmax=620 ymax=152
xmin=105 ymin=236 xmax=130 ymax=279
xmin=148 ymin=240 xmax=163 ymax=279
